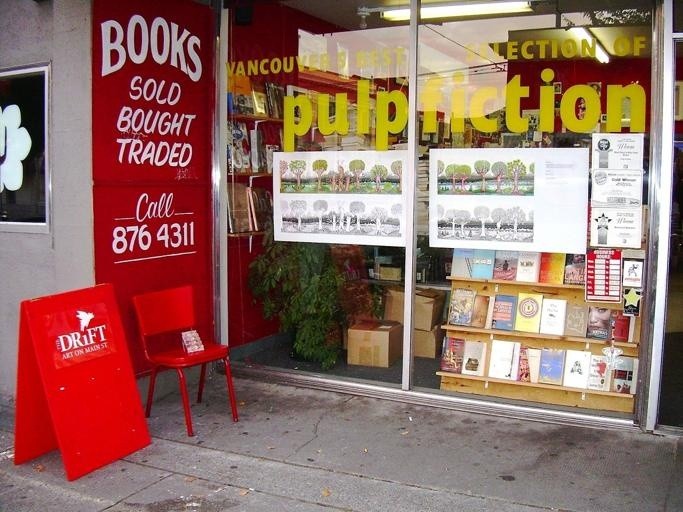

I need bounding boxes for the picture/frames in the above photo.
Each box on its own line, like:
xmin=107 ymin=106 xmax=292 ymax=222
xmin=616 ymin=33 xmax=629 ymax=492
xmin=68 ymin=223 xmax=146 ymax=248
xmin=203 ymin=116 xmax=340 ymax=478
xmin=286 ymin=85 xmax=336 ymax=122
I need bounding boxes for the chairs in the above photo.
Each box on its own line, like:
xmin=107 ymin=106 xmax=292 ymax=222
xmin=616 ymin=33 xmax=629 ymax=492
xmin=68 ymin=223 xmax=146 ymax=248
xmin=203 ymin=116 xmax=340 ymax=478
xmin=130 ymin=283 xmax=238 ymax=436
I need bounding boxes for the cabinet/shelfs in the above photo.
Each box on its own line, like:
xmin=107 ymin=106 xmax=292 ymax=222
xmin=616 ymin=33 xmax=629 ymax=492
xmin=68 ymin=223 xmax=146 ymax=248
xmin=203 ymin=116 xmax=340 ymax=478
xmin=228 ymin=113 xmax=284 ymax=239
xmin=439 ymin=249 xmax=649 ymax=415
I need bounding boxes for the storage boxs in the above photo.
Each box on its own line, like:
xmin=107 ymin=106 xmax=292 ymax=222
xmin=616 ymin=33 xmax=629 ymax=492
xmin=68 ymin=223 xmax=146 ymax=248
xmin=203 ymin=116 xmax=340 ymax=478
xmin=342 ymin=285 xmax=446 ymax=370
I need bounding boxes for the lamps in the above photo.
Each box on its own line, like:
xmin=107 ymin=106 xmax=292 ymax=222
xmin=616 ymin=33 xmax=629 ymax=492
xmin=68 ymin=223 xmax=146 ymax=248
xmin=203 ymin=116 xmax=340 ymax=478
xmin=379 ymin=1 xmax=536 ymax=22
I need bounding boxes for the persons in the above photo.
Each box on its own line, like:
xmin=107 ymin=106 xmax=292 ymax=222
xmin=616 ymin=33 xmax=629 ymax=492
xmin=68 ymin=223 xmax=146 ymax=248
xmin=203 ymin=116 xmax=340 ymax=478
xmin=565 ymin=254 xmax=585 ymax=284
xmin=587 ymin=306 xmax=610 ymax=339
xmin=503 ymin=260 xmax=508 ymax=270
